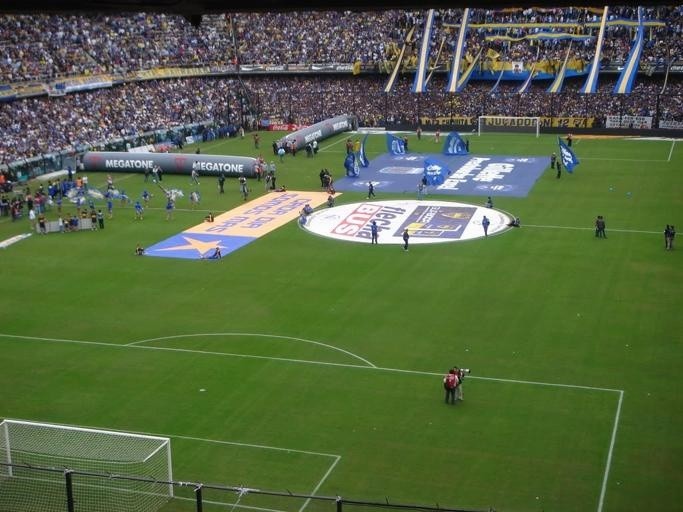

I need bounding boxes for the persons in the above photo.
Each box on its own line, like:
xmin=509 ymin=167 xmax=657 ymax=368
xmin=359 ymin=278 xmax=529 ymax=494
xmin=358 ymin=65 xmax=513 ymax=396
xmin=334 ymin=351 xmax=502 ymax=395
xmin=2 ymin=5 xmax=682 ymax=235
xmin=594 ymin=216 xmax=600 ymax=237
xmin=135 ymin=242 xmax=146 ymax=255
xmin=402 ymin=228 xmax=409 ymax=251
xmin=371 ymin=220 xmax=378 ymax=244
xmin=481 ymin=216 xmax=489 ymax=237
xmin=453 ymin=366 xmax=464 ymax=401
xmin=668 ymin=226 xmax=675 ymax=249
xmin=599 ymin=216 xmax=607 ymax=238
xmin=664 ymin=224 xmax=671 ymax=249
xmin=443 ymin=370 xmax=459 ymax=405
xmin=200 ymin=247 xmax=221 ymax=260
